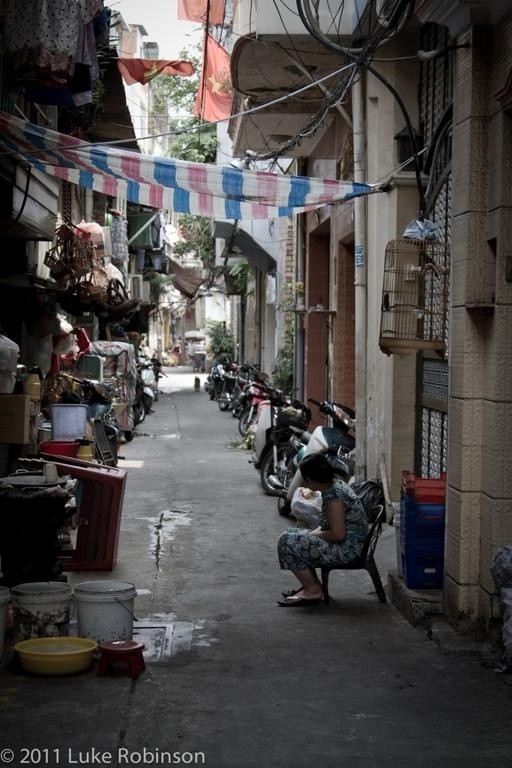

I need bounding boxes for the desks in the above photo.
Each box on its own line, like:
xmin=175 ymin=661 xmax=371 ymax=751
xmin=14 ymin=447 xmax=129 ymax=574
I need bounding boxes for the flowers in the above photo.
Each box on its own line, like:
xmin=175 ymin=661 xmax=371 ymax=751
xmin=292 ymin=281 xmax=306 ymax=296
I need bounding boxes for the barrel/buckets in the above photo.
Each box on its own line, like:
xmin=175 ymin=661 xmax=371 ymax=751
xmin=72 ymin=579 xmax=138 ymax=660
xmin=49 ymin=403 xmax=89 ymax=442
xmin=394 ymin=513 xmax=405 ymax=577
xmin=0 ymin=585 xmax=10 ymax=661
xmin=10 ymin=581 xmax=72 ymax=646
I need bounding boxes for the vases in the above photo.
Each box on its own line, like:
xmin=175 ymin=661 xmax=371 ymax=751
xmin=297 ymin=295 xmax=305 ymax=310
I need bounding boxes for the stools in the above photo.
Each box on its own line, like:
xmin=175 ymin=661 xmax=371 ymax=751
xmin=96 ymin=636 xmax=148 ymax=680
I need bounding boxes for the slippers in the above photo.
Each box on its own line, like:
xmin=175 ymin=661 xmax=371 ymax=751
xmin=279 ymin=588 xmax=300 ymax=600
xmin=276 ymin=596 xmax=318 ymax=607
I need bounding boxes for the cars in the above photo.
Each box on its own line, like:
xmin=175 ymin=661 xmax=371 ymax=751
xmin=192 ymin=345 xmax=206 ymax=358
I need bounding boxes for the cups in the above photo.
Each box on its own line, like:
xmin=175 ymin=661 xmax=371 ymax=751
xmin=43 ymin=463 xmax=58 ymax=483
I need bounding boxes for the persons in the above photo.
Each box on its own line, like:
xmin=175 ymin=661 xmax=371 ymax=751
xmin=276 ymin=457 xmax=369 ymax=610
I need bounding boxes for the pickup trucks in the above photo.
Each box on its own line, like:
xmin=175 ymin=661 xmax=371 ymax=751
xmin=167 ymin=344 xmax=182 ymax=358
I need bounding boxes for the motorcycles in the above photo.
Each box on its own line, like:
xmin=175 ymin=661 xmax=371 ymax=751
xmin=63 ymin=373 xmax=123 ymax=470
xmin=134 ymin=346 xmax=164 ymax=425
xmin=205 ymin=357 xmax=355 ymax=514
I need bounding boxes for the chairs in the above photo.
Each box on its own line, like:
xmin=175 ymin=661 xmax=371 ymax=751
xmin=317 ymin=502 xmax=391 ymax=605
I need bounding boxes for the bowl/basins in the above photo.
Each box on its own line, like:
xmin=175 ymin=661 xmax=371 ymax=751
xmin=14 ymin=637 xmax=98 ymax=675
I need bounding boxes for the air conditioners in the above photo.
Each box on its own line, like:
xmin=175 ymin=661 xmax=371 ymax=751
xmin=128 ymin=273 xmax=145 ymax=300
